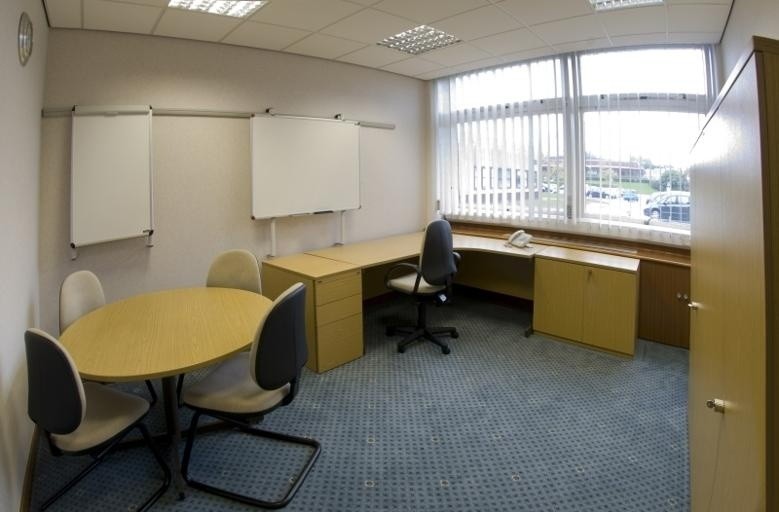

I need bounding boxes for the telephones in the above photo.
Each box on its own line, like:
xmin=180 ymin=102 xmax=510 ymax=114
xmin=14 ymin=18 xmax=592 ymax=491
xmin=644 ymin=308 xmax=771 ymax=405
xmin=504 ymin=230 xmax=533 ymax=248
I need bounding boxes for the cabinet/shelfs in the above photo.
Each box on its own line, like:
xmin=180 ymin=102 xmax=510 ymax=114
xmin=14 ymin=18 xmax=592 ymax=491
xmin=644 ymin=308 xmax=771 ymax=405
xmin=261 ymin=263 xmax=364 ymax=374
xmin=531 ymin=254 xmax=640 ymax=359
xmin=639 ymin=259 xmax=690 ymax=350
xmin=688 ymin=51 xmax=779 ymax=512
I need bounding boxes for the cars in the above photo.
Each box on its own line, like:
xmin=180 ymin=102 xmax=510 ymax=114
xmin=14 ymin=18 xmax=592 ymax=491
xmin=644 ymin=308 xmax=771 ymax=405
xmin=541 ymin=183 xmax=639 ymax=201
xmin=644 ymin=192 xmax=690 ymax=219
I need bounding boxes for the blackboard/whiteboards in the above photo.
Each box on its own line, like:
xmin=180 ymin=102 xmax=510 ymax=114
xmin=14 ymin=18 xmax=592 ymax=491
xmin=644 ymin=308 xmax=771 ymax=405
xmin=71 ymin=106 xmax=154 ymax=247
xmin=250 ymin=113 xmax=361 ymax=221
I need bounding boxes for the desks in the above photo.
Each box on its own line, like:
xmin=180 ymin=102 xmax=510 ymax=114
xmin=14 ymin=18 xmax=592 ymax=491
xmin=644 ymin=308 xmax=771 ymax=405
xmin=304 ymin=231 xmax=548 ymax=338
xmin=58 ymin=286 xmax=275 ymax=500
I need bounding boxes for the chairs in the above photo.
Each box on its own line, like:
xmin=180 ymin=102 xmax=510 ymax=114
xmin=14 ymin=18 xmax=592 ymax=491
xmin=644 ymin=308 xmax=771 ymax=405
xmin=182 ymin=281 xmax=322 ymax=509
xmin=176 ymin=248 xmax=264 ymax=407
xmin=23 ymin=329 xmax=172 ymax=512
xmin=383 ymin=220 xmax=462 ymax=354
xmin=59 ymin=270 xmax=158 ymax=409
